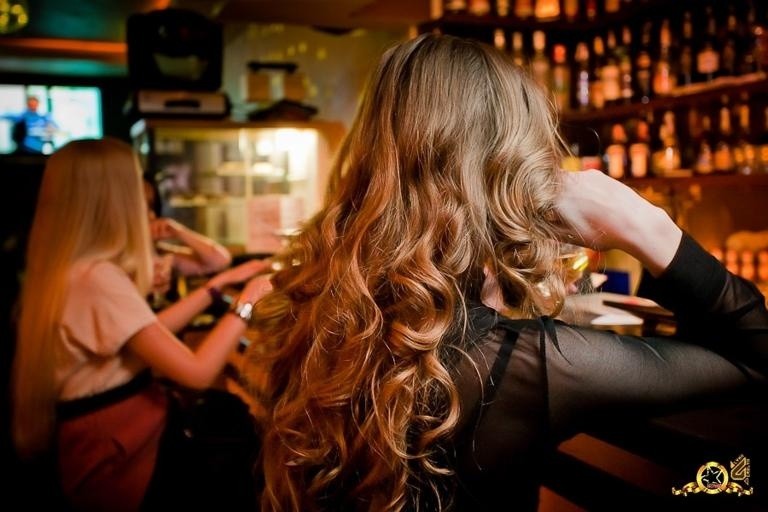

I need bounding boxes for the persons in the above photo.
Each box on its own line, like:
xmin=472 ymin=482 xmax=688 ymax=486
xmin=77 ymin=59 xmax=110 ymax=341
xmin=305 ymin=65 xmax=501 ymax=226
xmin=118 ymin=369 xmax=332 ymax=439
xmin=8 ymin=134 xmax=278 ymax=511
xmin=0 ymin=96 xmax=66 ymax=155
xmin=249 ymin=28 xmax=766 ymax=512
xmin=140 ymin=172 xmax=233 ymax=315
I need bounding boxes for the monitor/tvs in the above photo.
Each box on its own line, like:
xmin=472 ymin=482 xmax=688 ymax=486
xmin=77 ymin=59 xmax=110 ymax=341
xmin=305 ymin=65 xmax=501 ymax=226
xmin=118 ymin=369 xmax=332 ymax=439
xmin=127 ymin=11 xmax=223 ymax=88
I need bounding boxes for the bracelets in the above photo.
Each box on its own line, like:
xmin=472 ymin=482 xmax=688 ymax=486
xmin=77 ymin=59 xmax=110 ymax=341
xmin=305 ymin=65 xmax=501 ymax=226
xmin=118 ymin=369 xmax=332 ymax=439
xmin=202 ymin=279 xmax=222 ymax=299
xmin=227 ymin=296 xmax=252 ymax=319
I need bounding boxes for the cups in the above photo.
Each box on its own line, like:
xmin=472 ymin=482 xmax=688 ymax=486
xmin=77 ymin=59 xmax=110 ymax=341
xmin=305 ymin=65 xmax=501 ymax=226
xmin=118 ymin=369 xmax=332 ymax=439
xmin=283 ymin=73 xmax=307 ymax=101
xmin=247 ymin=74 xmax=271 ymax=102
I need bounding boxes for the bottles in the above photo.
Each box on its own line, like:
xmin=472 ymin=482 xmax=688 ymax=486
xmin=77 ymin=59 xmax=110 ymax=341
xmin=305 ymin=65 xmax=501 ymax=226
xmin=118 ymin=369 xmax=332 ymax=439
xmin=429 ymin=0 xmax=768 ymax=186
xmin=712 ymin=248 xmax=767 ymax=284
